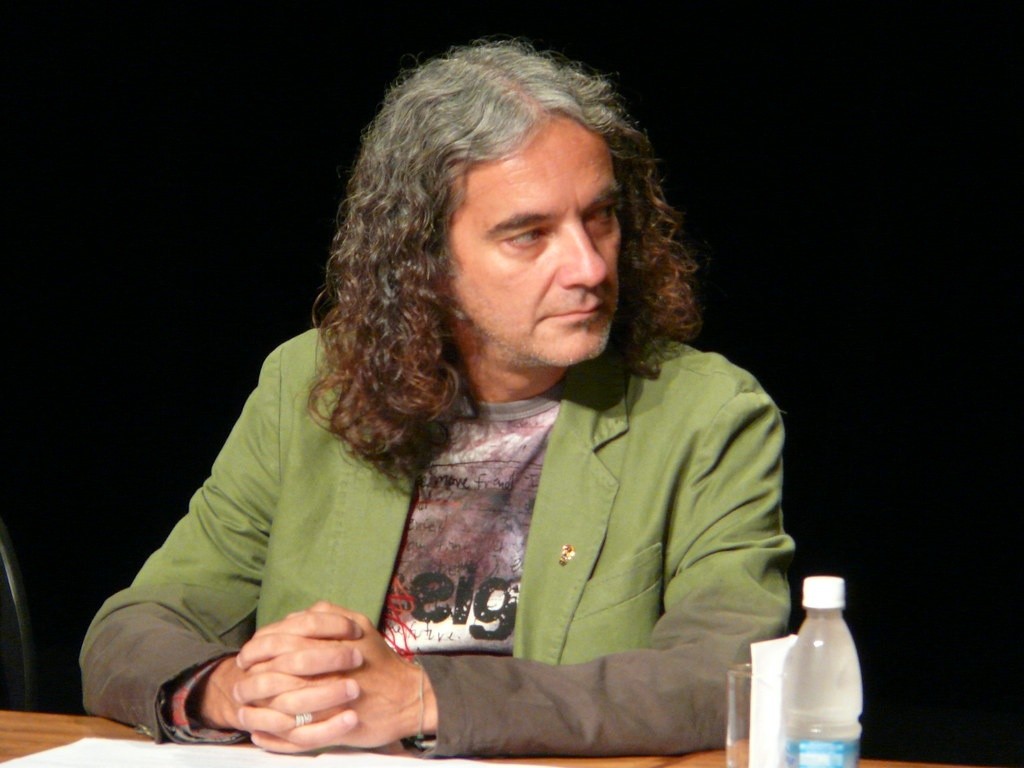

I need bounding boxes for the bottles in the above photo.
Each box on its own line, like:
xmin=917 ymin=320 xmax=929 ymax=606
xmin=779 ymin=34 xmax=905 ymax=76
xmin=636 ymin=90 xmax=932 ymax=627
xmin=777 ymin=576 xmax=863 ymax=767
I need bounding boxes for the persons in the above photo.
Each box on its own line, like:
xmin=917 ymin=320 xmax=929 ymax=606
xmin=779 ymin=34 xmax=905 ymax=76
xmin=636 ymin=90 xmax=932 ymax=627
xmin=81 ymin=40 xmax=796 ymax=755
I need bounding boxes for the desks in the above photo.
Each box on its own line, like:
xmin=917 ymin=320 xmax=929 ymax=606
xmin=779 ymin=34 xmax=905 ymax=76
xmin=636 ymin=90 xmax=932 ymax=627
xmin=0 ymin=710 xmax=997 ymax=768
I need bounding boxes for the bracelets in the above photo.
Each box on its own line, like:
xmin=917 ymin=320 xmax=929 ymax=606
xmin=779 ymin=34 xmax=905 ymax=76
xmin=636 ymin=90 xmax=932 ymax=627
xmin=417 ymin=666 xmax=426 ymax=739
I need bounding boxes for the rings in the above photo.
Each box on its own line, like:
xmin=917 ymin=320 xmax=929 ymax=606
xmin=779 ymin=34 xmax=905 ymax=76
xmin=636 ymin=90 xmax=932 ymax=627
xmin=296 ymin=713 xmax=312 ymax=728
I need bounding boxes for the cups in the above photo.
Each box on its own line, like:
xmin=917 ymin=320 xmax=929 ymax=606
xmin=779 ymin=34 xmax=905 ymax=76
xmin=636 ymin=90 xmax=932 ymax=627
xmin=727 ymin=661 xmax=752 ymax=768
